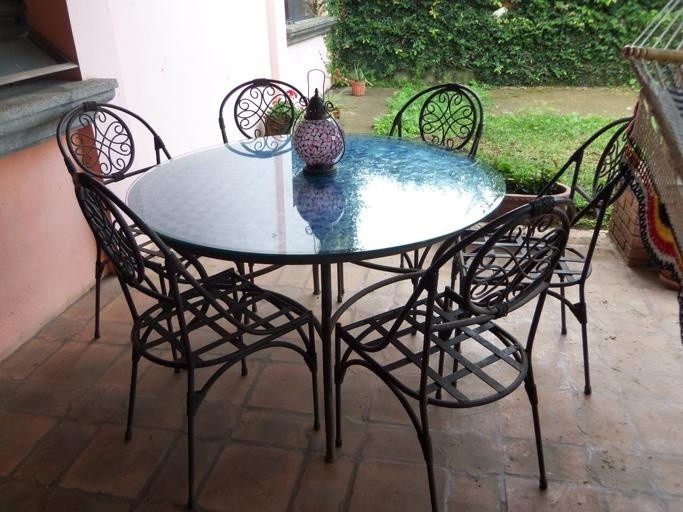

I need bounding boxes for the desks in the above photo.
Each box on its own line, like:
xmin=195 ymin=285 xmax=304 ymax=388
xmin=126 ymin=134 xmax=507 ymax=464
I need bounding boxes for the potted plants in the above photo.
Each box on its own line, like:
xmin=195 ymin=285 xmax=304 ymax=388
xmin=480 ymin=156 xmax=572 ymax=227
xmin=349 ymin=64 xmax=366 ymax=96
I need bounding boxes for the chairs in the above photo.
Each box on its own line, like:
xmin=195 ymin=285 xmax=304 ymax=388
xmin=337 ymin=82 xmax=482 ymax=335
xmin=69 ymin=174 xmax=321 ymax=512
xmin=217 ymin=81 xmax=319 ymax=295
xmin=444 ymin=119 xmax=642 ymax=395
xmin=334 ymin=206 xmax=571 ymax=512
xmin=57 ymin=100 xmax=188 ymax=340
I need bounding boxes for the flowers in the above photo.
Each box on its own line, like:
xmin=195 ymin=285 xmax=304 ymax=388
xmin=270 ymin=88 xmax=300 ymax=119
